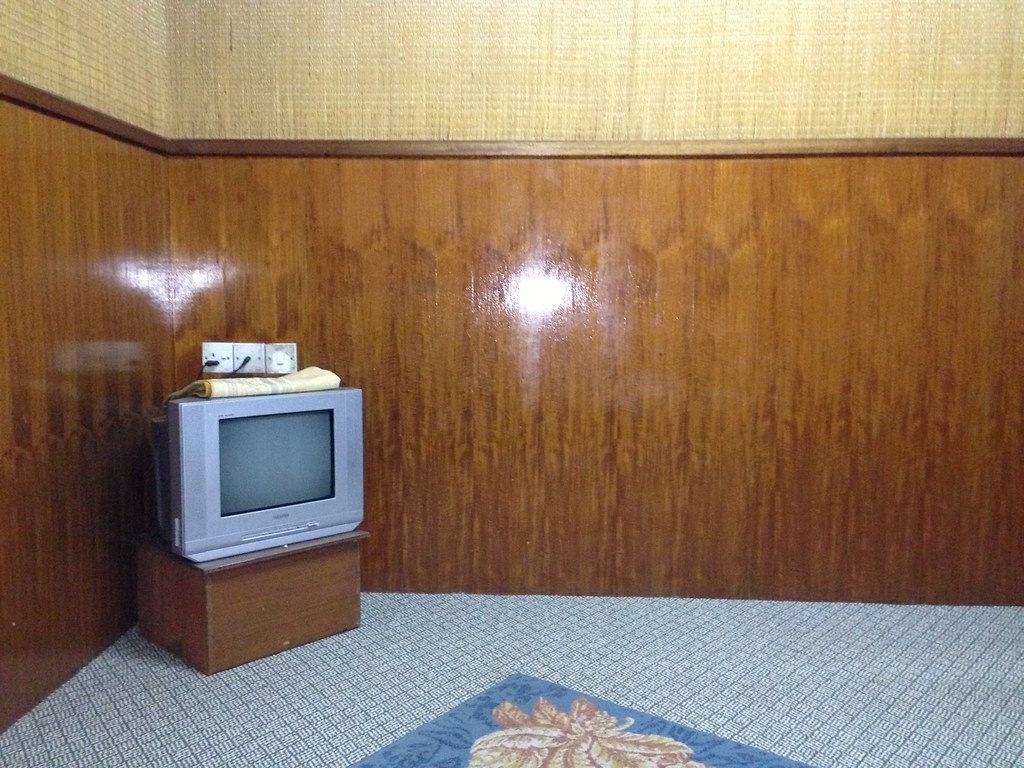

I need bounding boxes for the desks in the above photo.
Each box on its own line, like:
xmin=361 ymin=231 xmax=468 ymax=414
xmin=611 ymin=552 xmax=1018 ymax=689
xmin=126 ymin=530 xmax=371 ymax=676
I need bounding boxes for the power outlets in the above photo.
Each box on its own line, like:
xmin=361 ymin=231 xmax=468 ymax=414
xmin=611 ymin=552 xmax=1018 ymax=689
xmin=201 ymin=342 xmax=298 ymax=375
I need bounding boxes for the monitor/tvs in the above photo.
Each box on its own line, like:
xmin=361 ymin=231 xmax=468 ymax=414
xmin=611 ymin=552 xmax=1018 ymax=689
xmin=152 ymin=386 xmax=364 ymax=563
